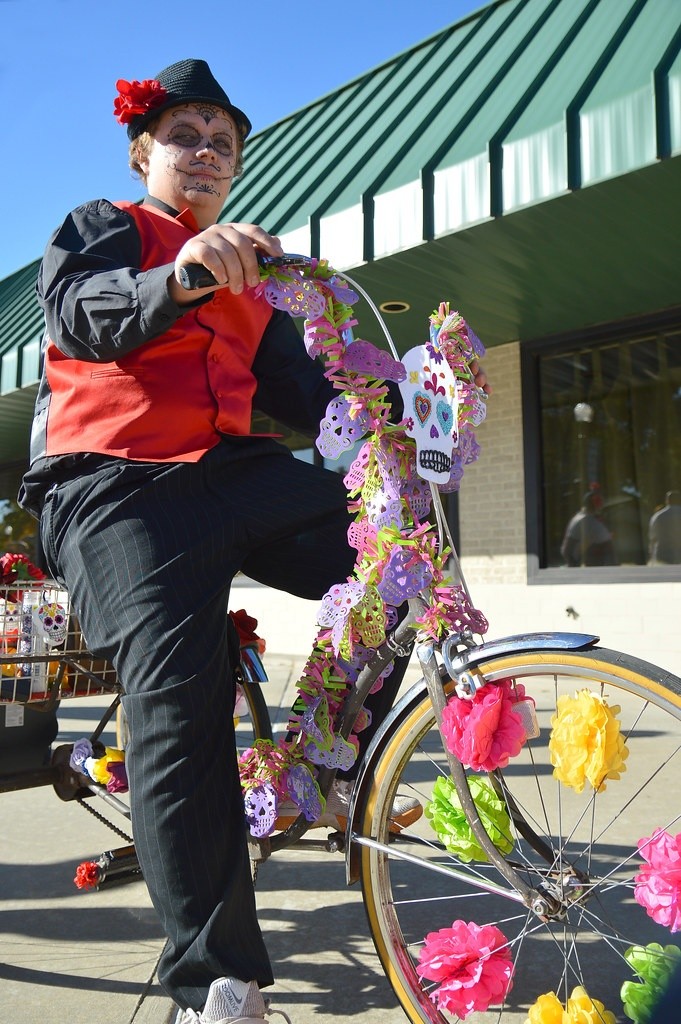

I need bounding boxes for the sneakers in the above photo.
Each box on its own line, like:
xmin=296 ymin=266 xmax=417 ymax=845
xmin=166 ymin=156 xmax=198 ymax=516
xmin=174 ymin=976 xmax=267 ymax=1024
xmin=274 ymin=778 xmax=423 ymax=834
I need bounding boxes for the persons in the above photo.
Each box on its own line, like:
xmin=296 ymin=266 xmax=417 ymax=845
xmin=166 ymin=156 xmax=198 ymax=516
xmin=559 ymin=483 xmax=681 ymax=566
xmin=27 ymin=57 xmax=494 ymax=1023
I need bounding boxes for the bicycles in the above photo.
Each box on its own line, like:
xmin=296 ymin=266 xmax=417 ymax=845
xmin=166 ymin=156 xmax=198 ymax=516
xmin=0 ymin=250 xmax=681 ymax=1024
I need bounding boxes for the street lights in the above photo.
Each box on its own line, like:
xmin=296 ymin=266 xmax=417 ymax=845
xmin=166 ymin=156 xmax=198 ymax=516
xmin=570 ymin=398 xmax=598 ymax=566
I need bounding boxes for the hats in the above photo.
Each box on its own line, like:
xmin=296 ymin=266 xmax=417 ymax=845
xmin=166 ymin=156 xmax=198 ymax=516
xmin=113 ymin=58 xmax=252 ymax=142
